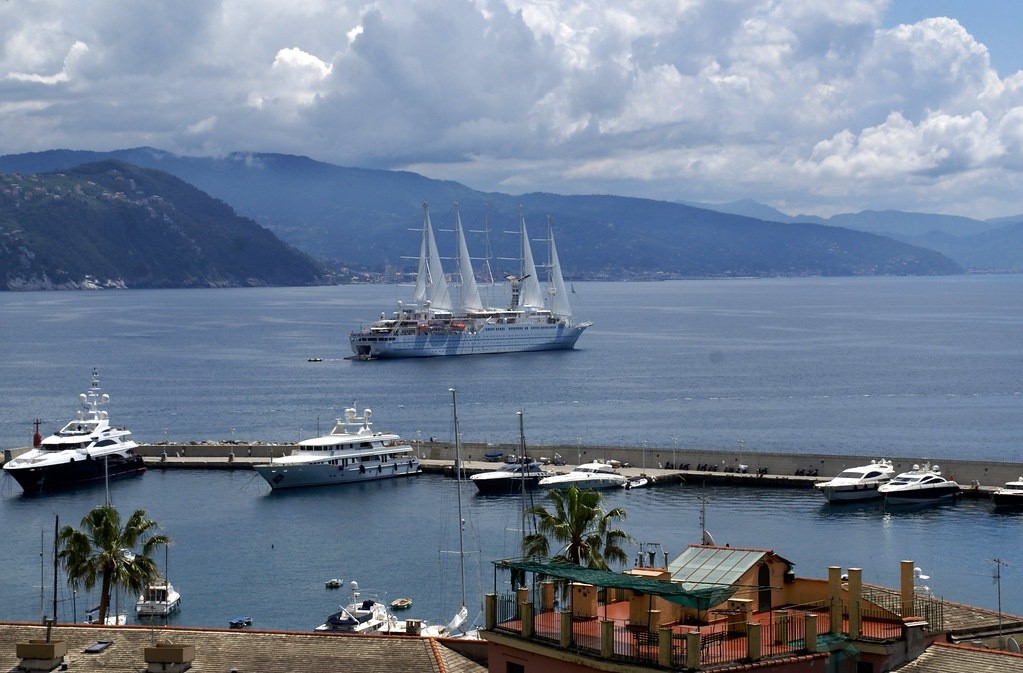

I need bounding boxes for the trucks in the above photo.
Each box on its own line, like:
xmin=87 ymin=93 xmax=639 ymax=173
xmin=540 ymin=453 xmax=567 ymax=465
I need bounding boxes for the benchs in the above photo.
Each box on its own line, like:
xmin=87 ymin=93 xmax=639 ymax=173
xmin=700 ymin=630 xmax=725 ymax=661
xmin=634 ymin=630 xmax=677 ymax=659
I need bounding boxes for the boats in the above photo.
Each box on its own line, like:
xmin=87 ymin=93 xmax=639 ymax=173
xmin=877 ymin=460 xmax=964 ymax=506
xmin=390 ymin=597 xmax=413 ymax=608
xmin=621 ymin=474 xmax=649 ymax=490
xmin=229 ymin=615 xmax=253 ymax=627
xmin=469 ymin=461 xmax=554 ymax=492
xmin=134 ymin=578 xmax=181 ymax=619
xmin=483 ymin=452 xmax=502 ymax=460
xmin=417 ymin=324 xmax=433 ymax=332
xmin=992 ymin=475 xmax=1023 ymax=512
xmin=312 ymin=580 xmax=398 ymax=634
xmin=324 ymin=578 xmax=343 ymax=588
xmin=539 ymin=460 xmax=626 ymax=489
xmin=815 ymin=459 xmax=893 ymax=505
xmin=3 ymin=366 xmax=147 ymax=492
xmin=450 ymin=323 xmax=464 ymax=329
xmin=251 ymin=397 xmax=422 ymax=489
xmin=82 ymin=602 xmax=128 ymax=627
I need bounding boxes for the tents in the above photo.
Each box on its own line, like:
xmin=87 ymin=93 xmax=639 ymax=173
xmin=491 ymin=555 xmax=739 ymax=633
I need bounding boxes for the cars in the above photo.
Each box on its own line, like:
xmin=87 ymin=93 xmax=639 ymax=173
xmin=503 ymin=454 xmax=517 ymax=464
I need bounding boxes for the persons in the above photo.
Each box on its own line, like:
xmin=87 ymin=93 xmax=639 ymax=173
xmin=430 ymin=436 xmax=437 ymax=442
xmin=765 ymin=465 xmax=768 ymax=474
xmin=666 ymin=460 xmax=669 ymax=469
xmin=468 ymin=453 xmax=472 ymax=463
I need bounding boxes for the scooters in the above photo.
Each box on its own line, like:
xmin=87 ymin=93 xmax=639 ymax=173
xmin=664 ymin=462 xmax=818 ymax=476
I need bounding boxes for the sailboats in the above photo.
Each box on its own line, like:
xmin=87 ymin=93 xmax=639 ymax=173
xmin=347 ymin=197 xmax=594 ymax=357
xmin=376 ymin=387 xmax=510 ymax=640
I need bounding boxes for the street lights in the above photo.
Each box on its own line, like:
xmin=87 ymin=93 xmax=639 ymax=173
xmin=738 ymin=439 xmax=744 ymax=475
xmin=641 ymin=440 xmax=648 ymax=468
xmin=671 ymin=437 xmax=678 ymax=469
